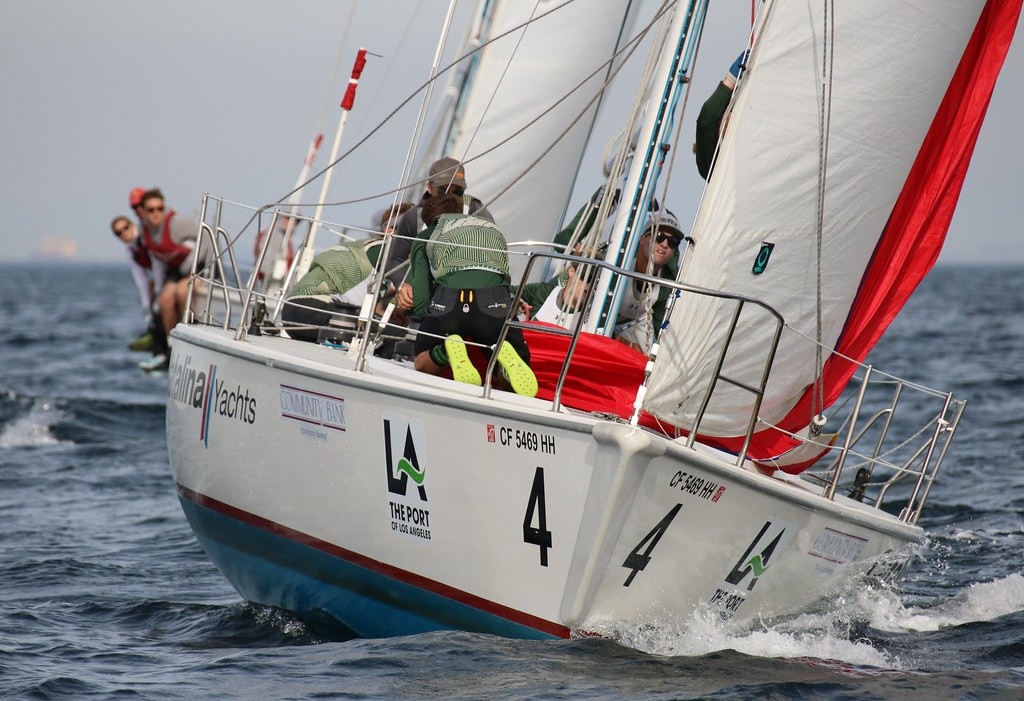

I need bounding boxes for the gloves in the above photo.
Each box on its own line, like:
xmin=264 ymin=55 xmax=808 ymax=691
xmin=727 ymin=49 xmax=750 ymax=85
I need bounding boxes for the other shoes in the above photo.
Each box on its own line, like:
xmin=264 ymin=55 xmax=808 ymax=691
xmin=139 ymin=353 xmax=169 ymax=370
xmin=444 ymin=334 xmax=482 ymax=386
xmin=128 ymin=334 xmax=156 ymax=351
xmin=496 ymin=340 xmax=538 ymax=397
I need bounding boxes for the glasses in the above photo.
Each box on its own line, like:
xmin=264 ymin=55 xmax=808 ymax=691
xmin=116 ymin=226 xmax=129 ymax=237
xmin=133 ymin=203 xmax=143 ymax=209
xmin=580 ymin=274 xmax=595 ymax=284
xmin=645 ymin=231 xmax=680 ymax=248
xmin=437 ymin=187 xmax=463 ymax=196
xmin=147 ymin=207 xmax=163 ymax=212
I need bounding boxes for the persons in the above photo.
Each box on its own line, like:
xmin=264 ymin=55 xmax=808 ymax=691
xmin=553 ymin=186 xmax=660 ymax=255
xmin=112 ymin=217 xmax=170 ymax=371
xmin=599 ymin=213 xmax=685 ymax=355
xmin=508 ymin=254 xmax=604 ymax=331
xmin=281 ymin=202 xmax=416 ymax=360
xmin=386 ymin=157 xmax=495 ymax=311
xmin=255 ymin=210 xmax=304 ymax=283
xmin=136 ymin=190 xmax=222 ymax=348
xmin=696 ymin=49 xmax=751 ymax=184
xmin=411 ymin=194 xmax=538 ymax=398
xmin=129 ymin=188 xmax=146 ymax=213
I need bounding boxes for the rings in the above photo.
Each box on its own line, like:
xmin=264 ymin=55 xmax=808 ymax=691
xmin=396 ymin=306 xmax=400 ymax=309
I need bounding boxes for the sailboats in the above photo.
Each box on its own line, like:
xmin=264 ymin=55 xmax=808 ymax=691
xmin=167 ymin=0 xmax=1023 ymax=672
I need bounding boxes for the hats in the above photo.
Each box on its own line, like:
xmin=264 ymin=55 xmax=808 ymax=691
xmin=429 ymin=156 xmax=467 ymax=190
xmin=130 ymin=188 xmax=145 ymax=205
xmin=570 ymin=252 xmax=605 ymax=276
xmin=641 ymin=213 xmax=684 ymax=240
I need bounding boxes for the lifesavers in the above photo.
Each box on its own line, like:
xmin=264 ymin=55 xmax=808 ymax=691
xmin=253 ymin=224 xmax=295 ymax=283
